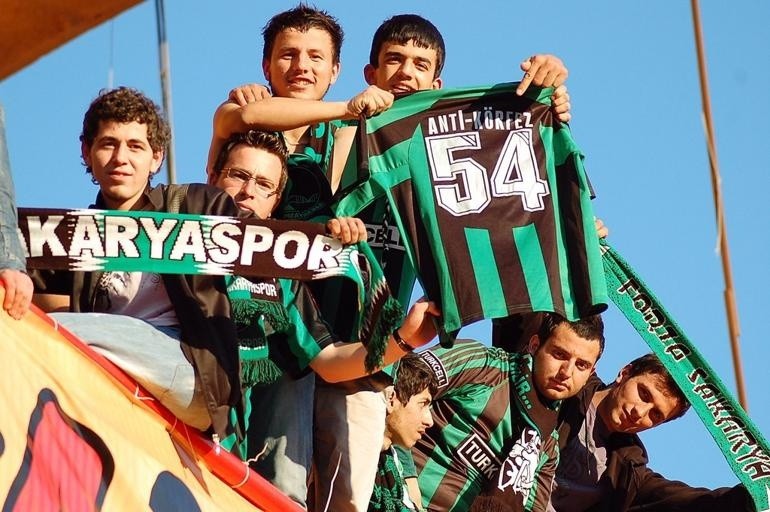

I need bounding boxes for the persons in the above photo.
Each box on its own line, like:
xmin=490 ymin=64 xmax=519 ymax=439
xmin=366 ymin=355 xmax=439 ymax=510
xmin=209 ymin=129 xmax=441 ymax=466
xmin=492 ymin=214 xmax=759 ymax=510
xmin=0 ymin=108 xmax=35 ymax=321
xmin=32 ymin=87 xmax=368 ymax=432
xmin=394 ymin=314 xmax=605 ymax=511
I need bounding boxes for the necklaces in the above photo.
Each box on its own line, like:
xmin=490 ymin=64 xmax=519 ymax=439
xmin=282 ymin=129 xmax=309 ymax=147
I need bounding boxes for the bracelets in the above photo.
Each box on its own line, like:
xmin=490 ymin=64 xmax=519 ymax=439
xmin=393 ymin=327 xmax=414 ymax=353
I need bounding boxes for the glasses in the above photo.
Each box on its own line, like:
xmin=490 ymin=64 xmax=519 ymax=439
xmin=221 ymin=167 xmax=279 ymax=199
